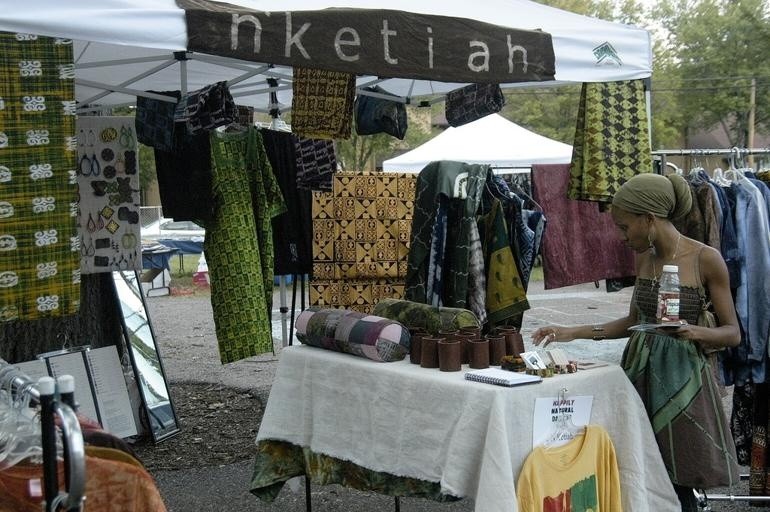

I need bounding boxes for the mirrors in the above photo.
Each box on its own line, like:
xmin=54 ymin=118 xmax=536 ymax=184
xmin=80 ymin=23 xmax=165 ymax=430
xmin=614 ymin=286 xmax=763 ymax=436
xmin=111 ymin=267 xmax=181 ymax=444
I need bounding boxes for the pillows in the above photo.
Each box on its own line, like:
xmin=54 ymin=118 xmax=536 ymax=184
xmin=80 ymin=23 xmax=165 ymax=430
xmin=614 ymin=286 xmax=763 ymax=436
xmin=292 ymin=298 xmax=483 ymax=362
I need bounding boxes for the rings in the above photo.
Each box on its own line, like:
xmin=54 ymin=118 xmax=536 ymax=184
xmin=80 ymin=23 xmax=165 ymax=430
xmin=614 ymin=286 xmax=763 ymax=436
xmin=550 ymin=332 xmax=555 ymax=341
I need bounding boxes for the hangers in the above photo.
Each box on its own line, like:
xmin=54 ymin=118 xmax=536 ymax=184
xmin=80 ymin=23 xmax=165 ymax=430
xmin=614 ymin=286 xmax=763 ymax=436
xmin=482 ymin=163 xmax=544 ymax=213
xmin=544 ymin=414 xmax=586 ymax=451
xmin=217 ymin=119 xmax=247 ymax=132
xmin=0 ymin=369 xmax=70 ymax=472
xmin=690 ymin=146 xmax=749 ymax=185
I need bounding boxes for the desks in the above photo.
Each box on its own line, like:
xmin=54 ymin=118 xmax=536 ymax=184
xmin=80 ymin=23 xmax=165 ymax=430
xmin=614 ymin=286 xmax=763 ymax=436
xmin=141 ymin=239 xmax=207 ymax=296
xmin=274 ymin=347 xmax=624 ymax=512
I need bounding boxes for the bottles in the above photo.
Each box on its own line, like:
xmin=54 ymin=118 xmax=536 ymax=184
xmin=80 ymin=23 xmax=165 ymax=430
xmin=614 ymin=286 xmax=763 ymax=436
xmin=655 ymin=265 xmax=682 ymax=324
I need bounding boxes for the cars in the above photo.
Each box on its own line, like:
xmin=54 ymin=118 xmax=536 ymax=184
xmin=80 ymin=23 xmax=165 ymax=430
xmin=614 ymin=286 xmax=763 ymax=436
xmin=140 ymin=218 xmax=206 ymax=239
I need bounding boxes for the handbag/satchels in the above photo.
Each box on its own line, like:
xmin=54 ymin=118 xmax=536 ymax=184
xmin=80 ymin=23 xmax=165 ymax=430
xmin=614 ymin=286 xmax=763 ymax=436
xmin=698 ymin=310 xmax=727 ymax=354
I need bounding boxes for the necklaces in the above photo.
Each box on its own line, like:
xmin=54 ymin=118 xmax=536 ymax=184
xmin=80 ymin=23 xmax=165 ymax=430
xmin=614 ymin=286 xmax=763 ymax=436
xmin=650 ymin=235 xmax=681 ymax=294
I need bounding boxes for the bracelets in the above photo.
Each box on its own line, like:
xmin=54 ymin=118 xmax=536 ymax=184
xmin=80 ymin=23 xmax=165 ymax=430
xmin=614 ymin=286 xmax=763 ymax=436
xmin=592 ymin=324 xmax=606 ymax=340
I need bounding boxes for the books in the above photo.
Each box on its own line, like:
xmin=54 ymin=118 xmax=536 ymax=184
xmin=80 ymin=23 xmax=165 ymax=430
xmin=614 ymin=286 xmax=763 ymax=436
xmin=464 ymin=367 xmax=542 ymax=387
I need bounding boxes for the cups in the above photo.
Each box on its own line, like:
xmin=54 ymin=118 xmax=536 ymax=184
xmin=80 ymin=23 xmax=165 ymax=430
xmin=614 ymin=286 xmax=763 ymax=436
xmin=408 ymin=324 xmax=525 ymax=374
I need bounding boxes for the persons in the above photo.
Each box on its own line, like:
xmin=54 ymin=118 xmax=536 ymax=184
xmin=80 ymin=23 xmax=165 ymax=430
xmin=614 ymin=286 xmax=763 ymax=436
xmin=722 ymin=157 xmax=738 ymax=169
xmin=530 ymin=172 xmax=741 ymax=512
